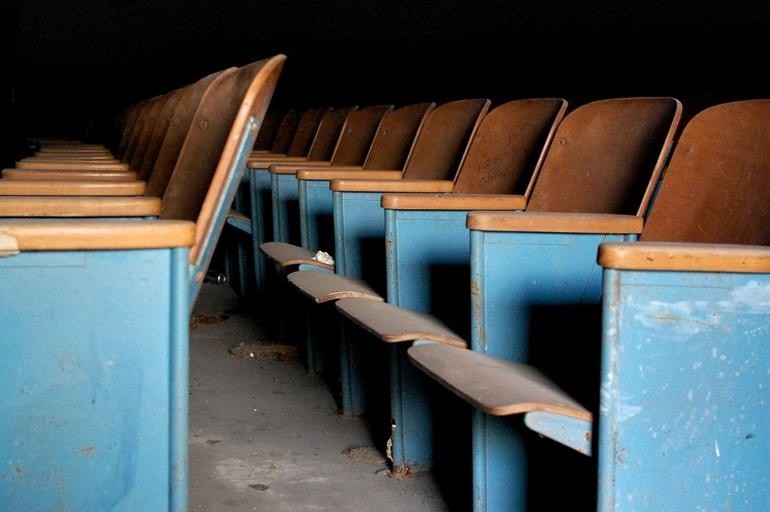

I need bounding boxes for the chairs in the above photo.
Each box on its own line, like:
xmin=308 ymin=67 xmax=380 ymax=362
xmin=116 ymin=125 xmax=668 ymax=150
xmin=0 ymin=53 xmax=290 ymax=512
xmin=210 ymin=98 xmax=770 ymax=511
